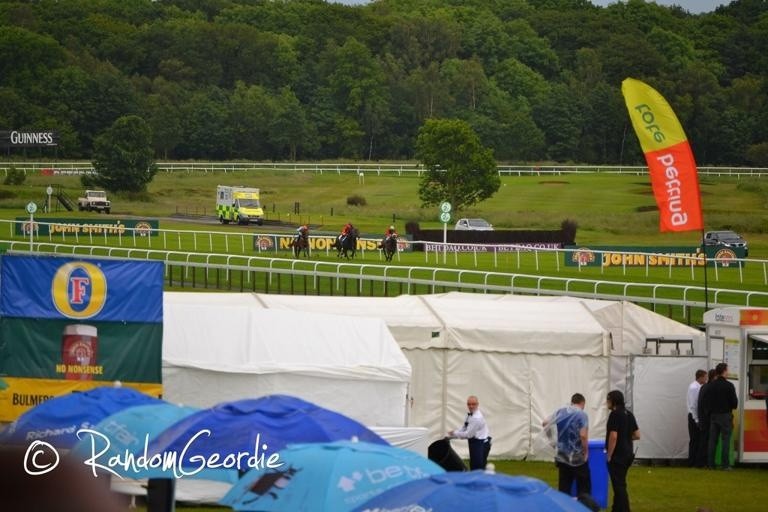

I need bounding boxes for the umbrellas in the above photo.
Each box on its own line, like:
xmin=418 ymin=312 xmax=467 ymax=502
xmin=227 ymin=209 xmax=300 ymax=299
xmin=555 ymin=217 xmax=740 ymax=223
xmin=132 ymin=391 xmax=399 ymax=474
xmin=65 ymin=401 xmax=208 ymax=476
xmin=212 ymin=439 xmax=450 ymax=512
xmin=0 ymin=383 xmax=182 ymax=452
xmin=347 ymin=466 xmax=598 ymax=512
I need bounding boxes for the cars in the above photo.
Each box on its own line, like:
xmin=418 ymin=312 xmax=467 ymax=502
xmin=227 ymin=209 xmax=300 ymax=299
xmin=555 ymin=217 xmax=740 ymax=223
xmin=454 ymin=218 xmax=494 ymax=231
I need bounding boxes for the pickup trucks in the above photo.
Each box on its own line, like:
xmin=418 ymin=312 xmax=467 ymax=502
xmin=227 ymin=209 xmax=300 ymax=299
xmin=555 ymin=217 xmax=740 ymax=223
xmin=78 ymin=190 xmax=110 ymax=214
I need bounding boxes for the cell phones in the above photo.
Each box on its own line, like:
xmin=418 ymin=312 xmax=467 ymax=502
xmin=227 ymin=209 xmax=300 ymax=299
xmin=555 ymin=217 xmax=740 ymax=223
xmin=147 ymin=477 xmax=175 ymax=512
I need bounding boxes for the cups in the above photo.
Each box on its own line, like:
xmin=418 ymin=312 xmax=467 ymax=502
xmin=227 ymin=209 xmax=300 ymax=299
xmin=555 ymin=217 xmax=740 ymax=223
xmin=60 ymin=324 xmax=98 ymax=381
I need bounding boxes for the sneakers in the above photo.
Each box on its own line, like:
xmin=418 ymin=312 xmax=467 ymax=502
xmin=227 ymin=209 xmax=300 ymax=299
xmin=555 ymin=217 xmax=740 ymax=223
xmin=686 ymin=461 xmax=734 ymax=471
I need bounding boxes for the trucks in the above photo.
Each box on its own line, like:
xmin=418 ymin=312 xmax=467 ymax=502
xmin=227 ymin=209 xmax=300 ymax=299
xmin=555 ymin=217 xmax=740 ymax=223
xmin=215 ymin=184 xmax=264 ymax=226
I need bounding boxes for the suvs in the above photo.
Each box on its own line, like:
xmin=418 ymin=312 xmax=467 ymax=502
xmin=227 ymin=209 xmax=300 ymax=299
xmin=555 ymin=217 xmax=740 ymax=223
xmin=700 ymin=229 xmax=748 ymax=257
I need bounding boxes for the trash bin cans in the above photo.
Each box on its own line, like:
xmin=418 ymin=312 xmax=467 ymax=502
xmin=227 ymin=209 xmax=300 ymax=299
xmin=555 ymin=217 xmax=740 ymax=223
xmin=706 ymin=415 xmax=735 ymax=466
xmin=570 ymin=440 xmax=609 ymax=509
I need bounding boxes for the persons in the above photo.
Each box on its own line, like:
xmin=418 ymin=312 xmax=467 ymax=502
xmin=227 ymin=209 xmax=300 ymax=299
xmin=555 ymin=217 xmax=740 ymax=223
xmin=685 ymin=369 xmax=708 ymax=467
xmin=539 ymin=391 xmax=592 ymax=502
xmin=336 ymin=222 xmax=354 ymax=243
xmin=601 ymin=389 xmax=642 ymax=511
xmin=697 ymin=367 xmax=719 ymax=467
xmin=296 ymin=224 xmax=308 ymax=238
xmin=703 ymin=361 xmax=738 ymax=471
xmin=383 ymin=225 xmax=398 ymax=249
xmin=444 ymin=393 xmax=492 ymax=472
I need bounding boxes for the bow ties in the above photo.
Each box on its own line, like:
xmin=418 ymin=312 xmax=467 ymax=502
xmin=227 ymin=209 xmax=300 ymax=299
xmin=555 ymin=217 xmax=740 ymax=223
xmin=467 ymin=412 xmax=473 ymax=416
